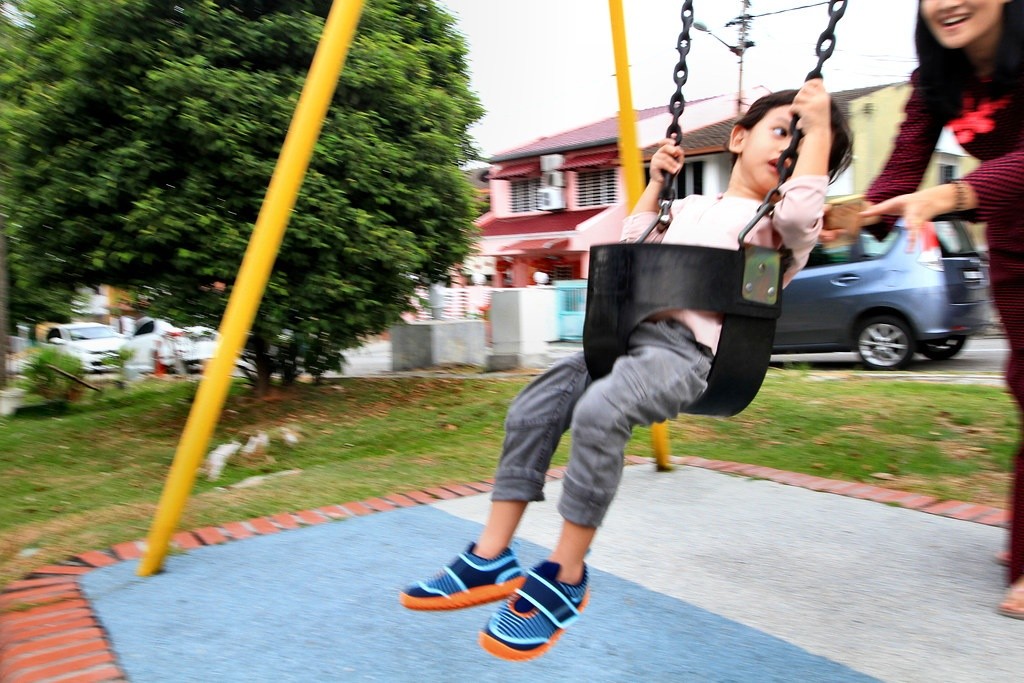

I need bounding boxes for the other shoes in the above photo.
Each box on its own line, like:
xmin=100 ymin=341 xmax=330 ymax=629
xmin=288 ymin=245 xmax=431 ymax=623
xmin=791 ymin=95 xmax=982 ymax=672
xmin=993 ymin=552 xmax=1013 ymax=567
xmin=998 ymin=579 xmax=1024 ymax=618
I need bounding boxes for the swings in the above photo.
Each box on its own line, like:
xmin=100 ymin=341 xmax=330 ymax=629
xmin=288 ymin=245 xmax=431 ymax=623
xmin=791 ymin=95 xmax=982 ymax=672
xmin=576 ymin=0 xmax=848 ymax=420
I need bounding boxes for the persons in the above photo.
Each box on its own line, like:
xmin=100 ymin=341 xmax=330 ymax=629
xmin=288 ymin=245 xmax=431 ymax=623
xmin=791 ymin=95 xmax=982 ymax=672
xmin=400 ymin=77 xmax=855 ymax=661
xmin=820 ymin=0 xmax=1024 ymax=619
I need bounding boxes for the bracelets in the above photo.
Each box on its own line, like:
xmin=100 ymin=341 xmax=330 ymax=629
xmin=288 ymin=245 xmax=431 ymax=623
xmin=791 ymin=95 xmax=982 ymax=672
xmin=949 ymin=177 xmax=968 ymax=211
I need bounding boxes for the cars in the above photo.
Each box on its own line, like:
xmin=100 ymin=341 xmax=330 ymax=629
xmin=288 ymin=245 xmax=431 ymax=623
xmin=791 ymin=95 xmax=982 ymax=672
xmin=46 ymin=315 xmax=221 ymax=374
xmin=769 ymin=218 xmax=994 ymax=372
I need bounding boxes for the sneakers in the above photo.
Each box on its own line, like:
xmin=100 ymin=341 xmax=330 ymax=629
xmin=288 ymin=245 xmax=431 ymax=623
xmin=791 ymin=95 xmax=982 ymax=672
xmin=476 ymin=559 xmax=587 ymax=660
xmin=398 ymin=541 xmax=526 ymax=610
